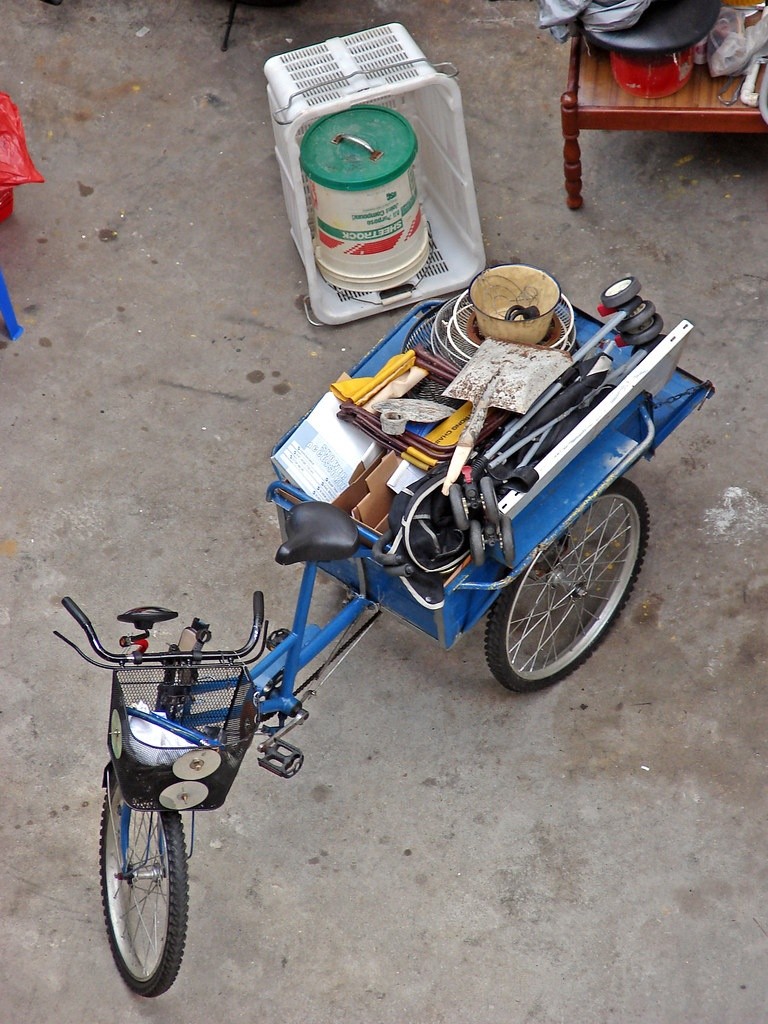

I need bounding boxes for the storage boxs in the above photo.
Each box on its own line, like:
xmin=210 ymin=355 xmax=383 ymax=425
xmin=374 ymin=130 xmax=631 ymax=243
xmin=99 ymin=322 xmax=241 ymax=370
xmin=279 ymin=448 xmax=387 ymax=514
xmin=349 ymin=449 xmax=403 ymax=535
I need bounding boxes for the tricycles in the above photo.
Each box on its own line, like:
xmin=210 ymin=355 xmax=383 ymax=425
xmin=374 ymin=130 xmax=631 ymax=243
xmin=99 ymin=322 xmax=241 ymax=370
xmin=52 ymin=303 xmax=720 ymax=998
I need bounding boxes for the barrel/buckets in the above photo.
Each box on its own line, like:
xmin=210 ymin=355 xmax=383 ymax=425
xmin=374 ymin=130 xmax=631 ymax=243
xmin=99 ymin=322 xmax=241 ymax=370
xmin=300 ymin=105 xmax=433 ymax=305
xmin=610 ymin=44 xmax=695 ymax=99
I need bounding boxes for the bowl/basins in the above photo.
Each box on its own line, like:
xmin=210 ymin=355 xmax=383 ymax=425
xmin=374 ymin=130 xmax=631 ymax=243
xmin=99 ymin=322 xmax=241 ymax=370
xmin=469 ymin=263 xmax=561 ymax=346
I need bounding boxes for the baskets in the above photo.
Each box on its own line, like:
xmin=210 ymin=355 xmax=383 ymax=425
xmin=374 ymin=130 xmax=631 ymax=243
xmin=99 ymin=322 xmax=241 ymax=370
xmin=106 ymin=664 xmax=260 ymax=812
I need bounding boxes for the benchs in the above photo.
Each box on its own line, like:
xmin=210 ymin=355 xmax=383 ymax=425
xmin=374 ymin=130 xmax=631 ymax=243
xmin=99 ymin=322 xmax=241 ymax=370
xmin=560 ymin=13 xmax=768 ymax=211
